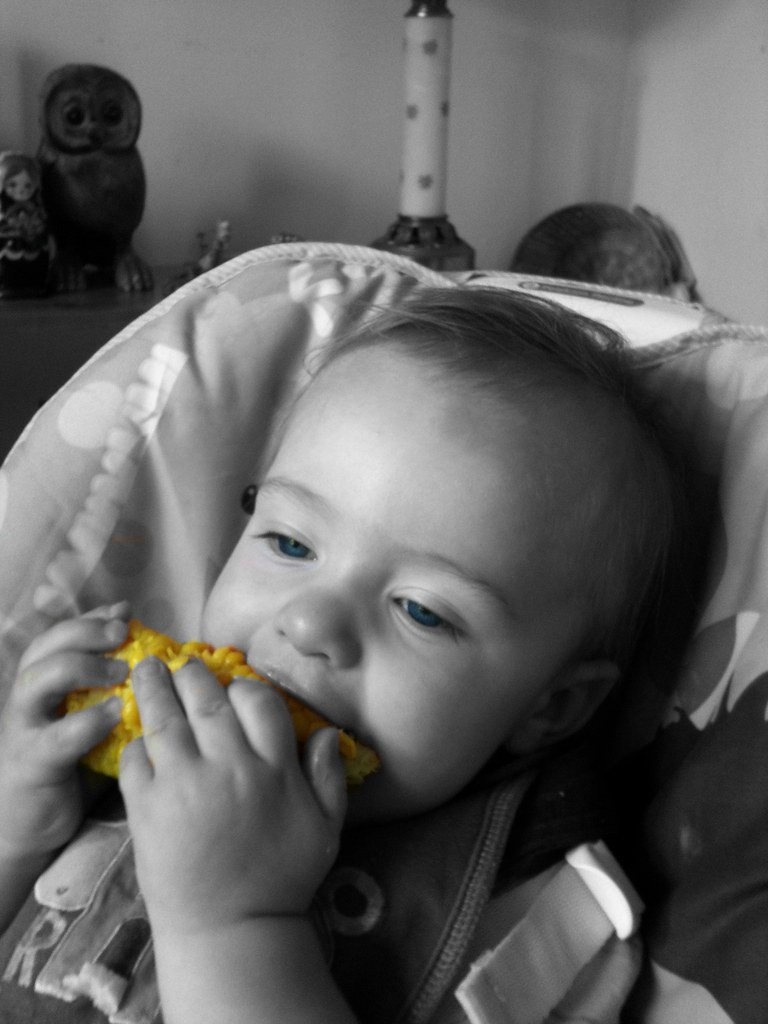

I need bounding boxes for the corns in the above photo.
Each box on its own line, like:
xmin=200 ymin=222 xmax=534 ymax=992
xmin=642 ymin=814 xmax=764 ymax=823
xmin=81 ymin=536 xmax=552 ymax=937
xmin=57 ymin=616 xmax=379 ymax=787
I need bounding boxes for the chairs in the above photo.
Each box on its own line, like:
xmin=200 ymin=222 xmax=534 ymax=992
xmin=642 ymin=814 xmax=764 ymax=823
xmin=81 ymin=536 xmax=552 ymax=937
xmin=0 ymin=243 xmax=768 ymax=1024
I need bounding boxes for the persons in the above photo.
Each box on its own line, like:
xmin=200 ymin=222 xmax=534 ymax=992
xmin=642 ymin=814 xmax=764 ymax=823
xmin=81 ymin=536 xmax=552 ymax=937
xmin=0 ymin=287 xmax=740 ymax=1024
